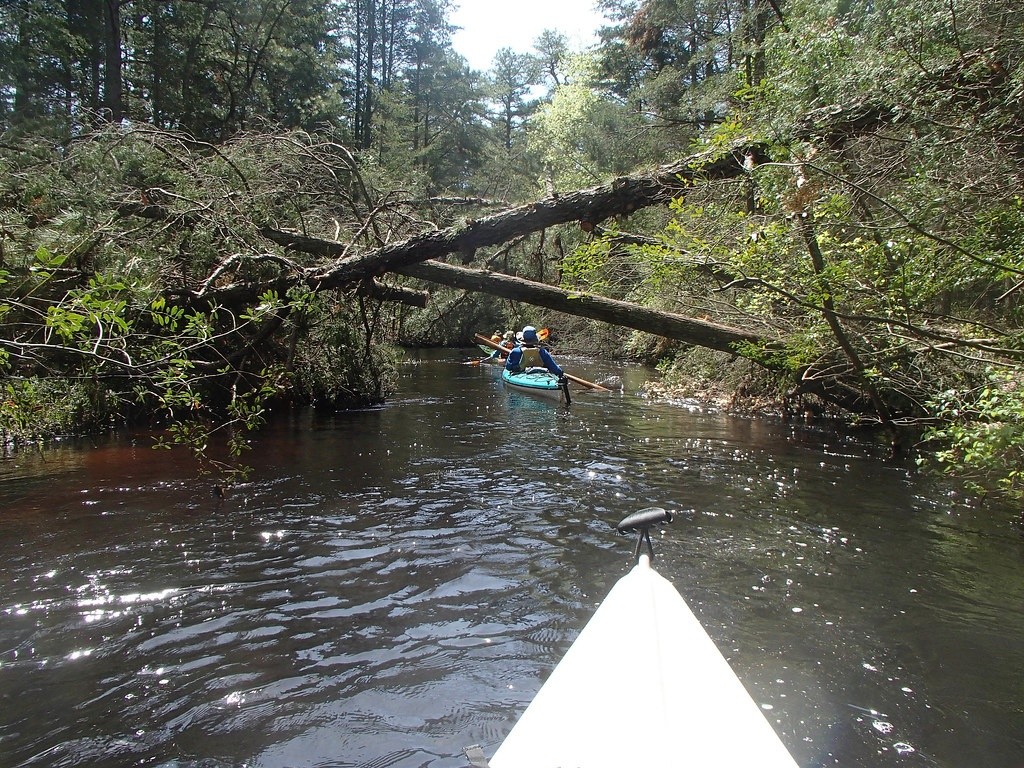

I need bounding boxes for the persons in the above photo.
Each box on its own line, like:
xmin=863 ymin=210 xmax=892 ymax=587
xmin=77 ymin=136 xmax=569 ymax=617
xmin=490 ymin=331 xmax=520 ymax=359
xmin=490 ymin=330 xmax=503 ymax=349
xmin=506 ymin=326 xmax=564 ymax=376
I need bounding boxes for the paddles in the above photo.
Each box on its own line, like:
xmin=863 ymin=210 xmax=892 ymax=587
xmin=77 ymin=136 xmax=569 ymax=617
xmin=474 ymin=332 xmax=607 ymax=390
xmin=463 ymin=329 xmax=549 ymax=365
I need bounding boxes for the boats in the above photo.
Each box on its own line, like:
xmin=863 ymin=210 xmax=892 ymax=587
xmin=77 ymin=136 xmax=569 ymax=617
xmin=501 ymin=367 xmax=564 ymax=402
xmin=479 ymin=344 xmax=507 ymax=365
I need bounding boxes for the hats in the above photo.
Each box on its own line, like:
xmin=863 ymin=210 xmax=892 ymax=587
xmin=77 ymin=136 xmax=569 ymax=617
xmin=502 ymin=330 xmax=516 ymax=339
xmin=516 ymin=325 xmax=540 ymax=345
xmin=494 ymin=330 xmax=502 ymax=335
